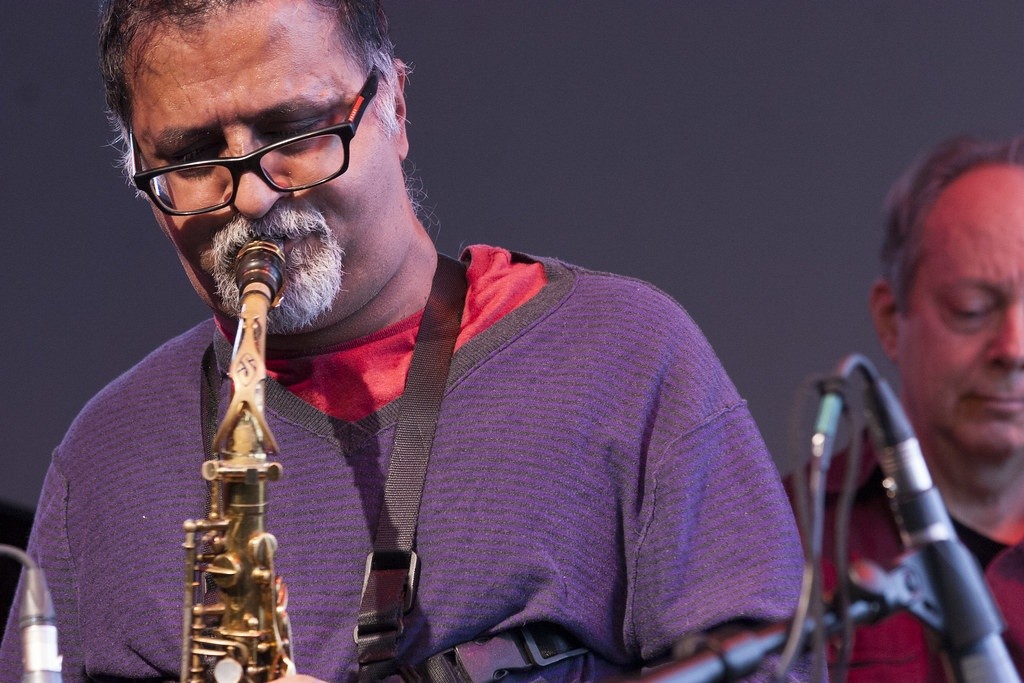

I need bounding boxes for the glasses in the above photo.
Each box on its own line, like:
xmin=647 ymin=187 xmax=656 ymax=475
xmin=130 ymin=66 xmax=380 ymax=215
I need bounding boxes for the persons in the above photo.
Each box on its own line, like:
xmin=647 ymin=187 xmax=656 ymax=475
xmin=0 ymin=0 xmax=828 ymax=683
xmin=783 ymin=135 xmax=1024 ymax=683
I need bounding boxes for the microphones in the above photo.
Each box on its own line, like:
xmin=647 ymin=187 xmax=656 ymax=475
xmin=864 ymin=382 xmax=1022 ymax=683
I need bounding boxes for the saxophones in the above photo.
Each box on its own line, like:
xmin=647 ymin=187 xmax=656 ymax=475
xmin=177 ymin=234 xmax=298 ymax=683
